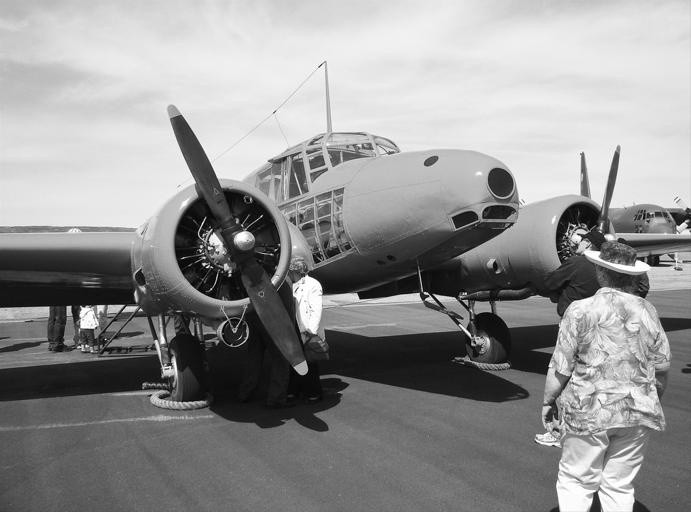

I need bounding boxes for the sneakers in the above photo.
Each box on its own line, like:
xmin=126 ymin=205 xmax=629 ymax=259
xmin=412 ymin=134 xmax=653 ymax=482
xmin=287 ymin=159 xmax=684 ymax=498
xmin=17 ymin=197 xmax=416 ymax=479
xmin=48 ymin=344 xmax=97 ymax=354
xmin=535 ymin=432 xmax=561 ymax=449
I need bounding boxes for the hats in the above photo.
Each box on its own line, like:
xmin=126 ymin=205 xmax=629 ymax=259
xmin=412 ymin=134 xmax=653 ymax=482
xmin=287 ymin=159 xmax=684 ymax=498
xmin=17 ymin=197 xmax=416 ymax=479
xmin=577 ymin=230 xmax=650 ymax=276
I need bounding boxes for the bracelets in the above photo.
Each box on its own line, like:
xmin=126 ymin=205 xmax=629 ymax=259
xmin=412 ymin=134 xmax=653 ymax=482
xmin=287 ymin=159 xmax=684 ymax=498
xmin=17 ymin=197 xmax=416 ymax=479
xmin=544 ymin=403 xmax=556 ymax=406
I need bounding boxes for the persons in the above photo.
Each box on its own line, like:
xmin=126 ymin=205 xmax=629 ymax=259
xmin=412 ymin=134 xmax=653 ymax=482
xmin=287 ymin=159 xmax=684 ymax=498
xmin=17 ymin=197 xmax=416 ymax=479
xmin=634 ymin=274 xmax=650 ymax=297
xmin=287 ymin=256 xmax=327 ymax=404
xmin=72 ymin=305 xmax=89 ymax=349
xmin=235 ymin=280 xmax=296 ymax=410
xmin=534 ymin=232 xmax=606 ymax=448
xmin=79 ymin=300 xmax=100 ymax=354
xmin=48 ymin=305 xmax=68 ymax=352
xmin=541 ymin=241 xmax=671 ymax=512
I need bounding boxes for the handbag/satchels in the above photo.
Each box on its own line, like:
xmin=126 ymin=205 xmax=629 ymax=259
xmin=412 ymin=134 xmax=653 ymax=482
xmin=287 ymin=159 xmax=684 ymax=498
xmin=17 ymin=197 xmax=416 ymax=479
xmin=302 ymin=334 xmax=330 ymax=363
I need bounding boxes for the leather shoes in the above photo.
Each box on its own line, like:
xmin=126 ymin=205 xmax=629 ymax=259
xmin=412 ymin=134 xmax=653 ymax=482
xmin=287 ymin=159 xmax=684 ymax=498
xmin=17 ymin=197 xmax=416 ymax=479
xmin=235 ymin=391 xmax=322 ymax=408
